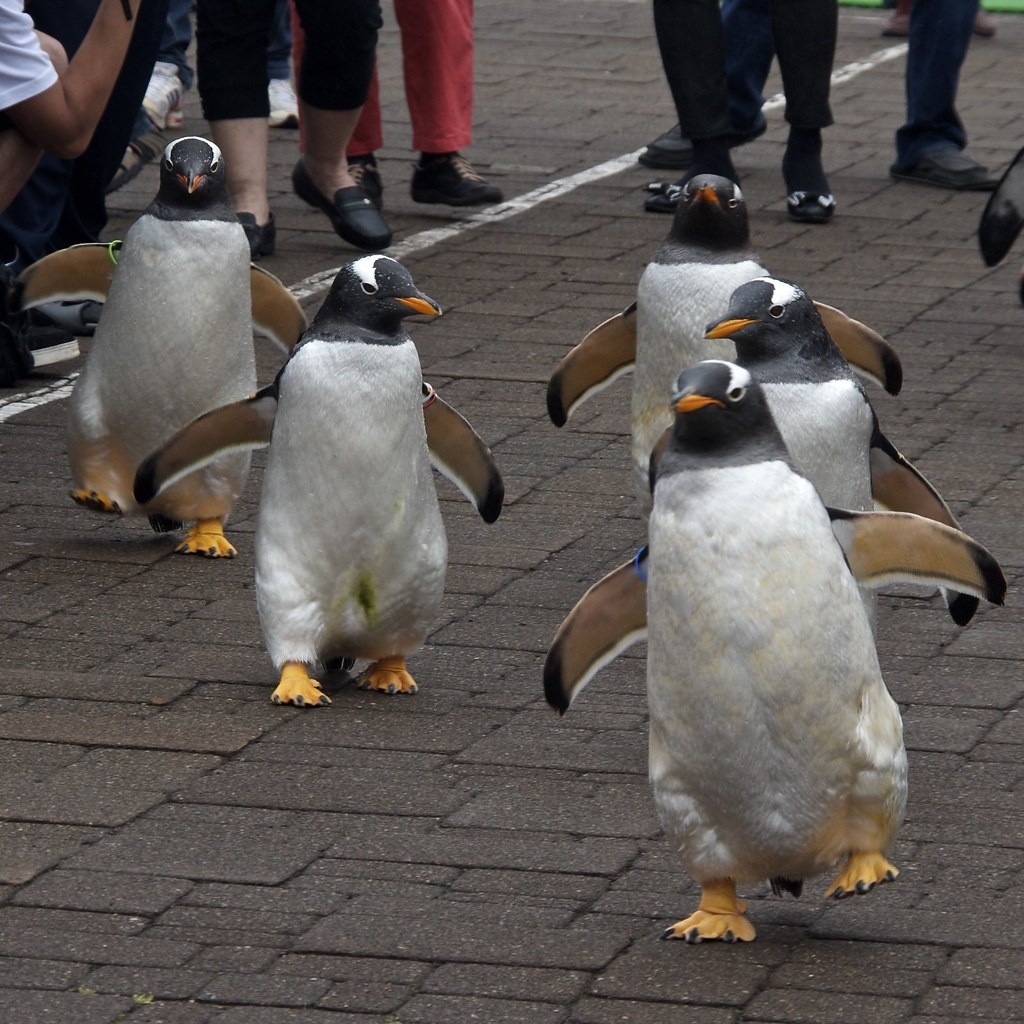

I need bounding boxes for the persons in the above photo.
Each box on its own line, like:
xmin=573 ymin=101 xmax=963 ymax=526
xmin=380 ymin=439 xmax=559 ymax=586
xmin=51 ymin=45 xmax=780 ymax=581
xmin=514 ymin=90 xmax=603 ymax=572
xmin=1 ymin=0 xmax=502 ymax=385
xmin=637 ymin=1 xmax=992 ymax=222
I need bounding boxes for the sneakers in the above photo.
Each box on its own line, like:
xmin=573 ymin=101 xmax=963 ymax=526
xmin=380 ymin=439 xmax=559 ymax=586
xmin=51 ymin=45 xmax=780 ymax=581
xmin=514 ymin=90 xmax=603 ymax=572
xmin=410 ymin=153 xmax=504 ymax=207
xmin=345 ymin=153 xmax=383 ymax=211
xmin=139 ymin=60 xmax=183 ymax=130
xmin=268 ymin=78 xmax=300 ymax=127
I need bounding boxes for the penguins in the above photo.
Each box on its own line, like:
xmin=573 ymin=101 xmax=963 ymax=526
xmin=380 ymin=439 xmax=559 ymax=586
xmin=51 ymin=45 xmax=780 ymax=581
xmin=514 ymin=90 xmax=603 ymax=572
xmin=540 ymin=173 xmax=1010 ymax=948
xmin=8 ymin=139 xmax=504 ymax=706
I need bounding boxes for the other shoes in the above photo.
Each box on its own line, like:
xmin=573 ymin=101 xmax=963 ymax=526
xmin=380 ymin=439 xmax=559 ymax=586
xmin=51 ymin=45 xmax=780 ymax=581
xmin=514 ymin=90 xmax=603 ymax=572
xmin=639 ymin=110 xmax=767 ymax=169
xmin=889 ymin=144 xmax=1000 ymax=189
xmin=293 ymin=159 xmax=392 ymax=252
xmin=973 ymin=12 xmax=996 ymax=36
xmin=782 ymin=147 xmax=835 ymax=222
xmin=23 ymin=330 xmax=79 ymax=367
xmin=643 ymin=167 xmax=743 ymax=215
xmin=881 ymin=0 xmax=912 ymax=36
xmin=35 ymin=298 xmax=104 ymax=328
xmin=234 ymin=211 xmax=276 ymax=261
xmin=103 ymin=108 xmax=167 ymax=196
